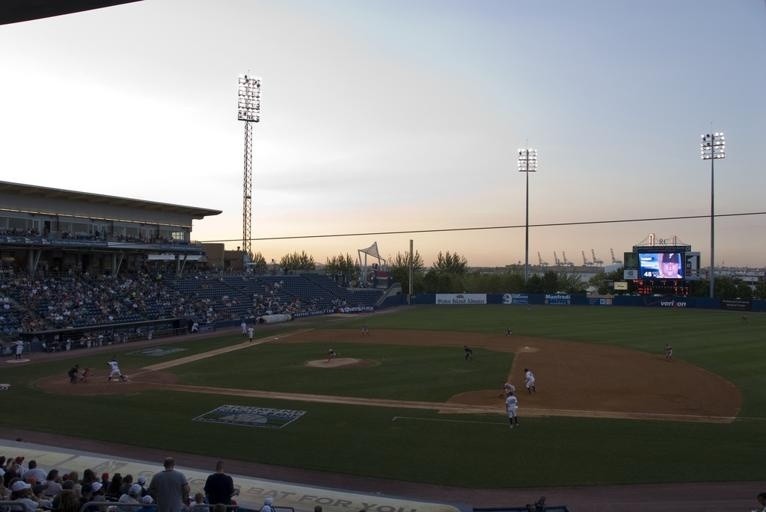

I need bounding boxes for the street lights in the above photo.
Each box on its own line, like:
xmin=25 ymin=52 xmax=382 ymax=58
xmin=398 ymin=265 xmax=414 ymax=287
xmin=236 ymin=74 xmax=262 ymax=255
xmin=699 ymin=131 xmax=727 ymax=299
xmin=516 ymin=148 xmax=540 ymax=283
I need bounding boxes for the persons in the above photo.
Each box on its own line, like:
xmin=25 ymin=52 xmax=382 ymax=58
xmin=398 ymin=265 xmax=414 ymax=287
xmin=360 ymin=325 xmax=369 ymax=337
xmin=67 ymin=364 xmax=80 ymax=384
xmin=112 ymin=265 xmax=238 ymax=340
xmin=248 ymin=325 xmax=255 ymax=342
xmin=2 ymin=228 xmax=196 ymax=247
xmin=498 ymin=379 xmax=521 ymax=405
xmin=504 ymin=391 xmax=520 ymax=429
xmin=240 ymin=319 xmax=247 ymax=336
xmin=503 ymin=327 xmax=513 ymax=336
xmin=663 ymin=342 xmax=674 ymax=360
xmin=521 ymin=367 xmax=537 ymax=395
xmin=328 ymin=348 xmax=337 ymax=362
xmin=260 ymin=497 xmax=273 ymax=511
xmin=231 ymin=265 xmax=374 ymax=317
xmin=656 ymin=253 xmax=682 ymax=278
xmin=80 ymin=365 xmax=93 ymax=383
xmin=1 ymin=456 xmax=239 ymax=511
xmin=2 ymin=261 xmax=123 ymax=360
xmin=463 ymin=345 xmax=473 ymax=361
xmin=106 ymin=358 xmax=126 ymax=383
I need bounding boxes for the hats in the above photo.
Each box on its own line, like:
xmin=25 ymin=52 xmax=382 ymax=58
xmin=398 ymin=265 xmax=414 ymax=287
xmin=264 ymin=497 xmax=273 ymax=504
xmin=261 ymin=505 xmax=272 ymax=512
xmin=138 ymin=477 xmax=145 ymax=484
xmin=143 ymin=495 xmax=154 ymax=503
xmin=12 ymin=480 xmax=31 ymax=491
xmin=91 ymin=481 xmax=103 ymax=492
xmin=0 ymin=467 xmax=5 ymax=476
xmin=128 ymin=483 xmax=142 ymax=494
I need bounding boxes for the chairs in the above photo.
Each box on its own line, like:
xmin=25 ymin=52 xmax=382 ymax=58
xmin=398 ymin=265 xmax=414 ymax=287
xmin=0 ymin=270 xmax=202 ymax=346
xmin=203 ymin=272 xmax=382 ymax=319
xmin=0 ymin=225 xmax=205 ymax=251
